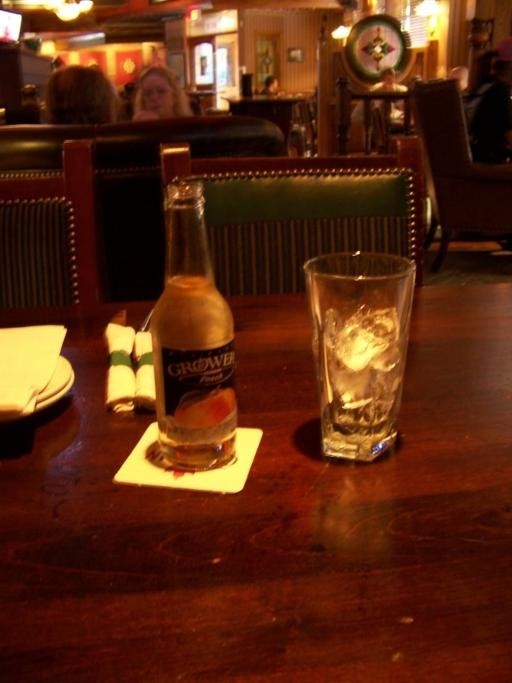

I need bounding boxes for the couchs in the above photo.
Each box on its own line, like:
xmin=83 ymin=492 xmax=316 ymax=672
xmin=1 ymin=115 xmax=311 ymax=302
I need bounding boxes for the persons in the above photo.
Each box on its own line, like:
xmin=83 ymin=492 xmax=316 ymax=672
xmin=131 ymin=65 xmax=191 ymax=121
xmin=19 ymin=83 xmax=46 ymax=120
xmin=48 ymin=64 xmax=114 ymax=122
xmin=262 ymin=73 xmax=278 ymax=95
xmin=370 ymin=66 xmax=408 ymax=119
xmin=466 ymin=37 xmax=511 ymax=163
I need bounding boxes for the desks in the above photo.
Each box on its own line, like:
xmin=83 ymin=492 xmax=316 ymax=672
xmin=219 ymin=96 xmax=307 ymax=154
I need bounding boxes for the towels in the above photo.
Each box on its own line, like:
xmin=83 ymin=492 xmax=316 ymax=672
xmin=100 ymin=320 xmax=137 ymax=416
xmin=134 ymin=330 xmax=162 ymax=414
xmin=1 ymin=323 xmax=69 ymax=424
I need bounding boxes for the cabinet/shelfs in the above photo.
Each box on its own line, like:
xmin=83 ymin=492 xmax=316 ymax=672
xmin=0 ymin=45 xmax=53 ymax=107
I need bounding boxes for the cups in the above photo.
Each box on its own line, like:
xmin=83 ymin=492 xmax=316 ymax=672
xmin=305 ymin=252 xmax=415 ymax=459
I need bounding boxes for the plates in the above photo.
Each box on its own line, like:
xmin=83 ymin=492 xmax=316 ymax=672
xmin=36 ymin=370 xmax=75 ymax=410
xmin=36 ymin=356 xmax=71 ymax=404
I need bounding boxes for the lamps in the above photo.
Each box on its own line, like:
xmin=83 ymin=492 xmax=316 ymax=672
xmin=41 ymin=0 xmax=95 ymax=23
xmin=414 ymin=0 xmax=444 ymax=36
xmin=330 ymin=23 xmax=351 ymax=42
xmin=468 ymin=16 xmax=493 ymax=50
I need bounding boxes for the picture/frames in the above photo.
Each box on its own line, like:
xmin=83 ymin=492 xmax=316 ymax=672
xmin=403 ymin=51 xmax=425 ymax=85
xmin=286 ymin=46 xmax=306 ymax=64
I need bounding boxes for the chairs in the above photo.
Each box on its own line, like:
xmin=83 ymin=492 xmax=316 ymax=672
xmin=336 ymin=74 xmax=415 ymax=155
xmin=1 ymin=133 xmax=106 ymax=309
xmin=4 ymin=103 xmax=41 ymax=124
xmin=306 ymin=93 xmax=320 ymax=157
xmin=159 ymin=134 xmax=428 ymax=301
xmin=414 ymin=78 xmax=511 ymax=275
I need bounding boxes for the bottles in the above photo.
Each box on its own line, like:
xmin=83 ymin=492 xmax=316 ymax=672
xmin=149 ymin=181 xmax=237 ymax=472
